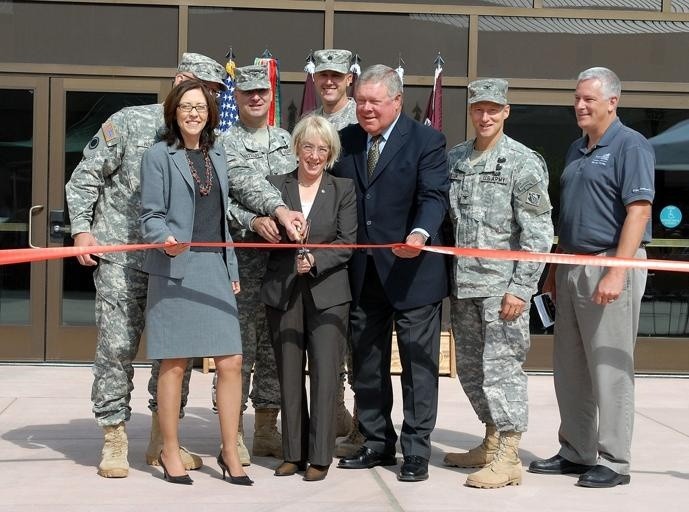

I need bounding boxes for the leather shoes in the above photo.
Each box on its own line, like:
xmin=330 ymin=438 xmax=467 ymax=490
xmin=529 ymin=454 xmax=596 ymax=473
xmin=578 ymin=465 xmax=629 ymax=487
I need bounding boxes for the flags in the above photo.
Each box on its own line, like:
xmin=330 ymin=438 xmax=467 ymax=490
xmin=216 ymin=60 xmax=240 ymax=132
xmin=254 ymin=57 xmax=281 ymax=128
xmin=423 ymin=67 xmax=442 ymax=133
xmin=350 ymin=64 xmax=360 ymax=98
xmin=301 ymin=61 xmax=317 ymax=114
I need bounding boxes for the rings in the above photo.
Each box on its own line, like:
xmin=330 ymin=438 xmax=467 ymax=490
xmin=609 ymin=300 xmax=614 ymax=302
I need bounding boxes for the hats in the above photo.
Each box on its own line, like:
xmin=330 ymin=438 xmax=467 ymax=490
xmin=177 ymin=53 xmax=229 ymax=92
xmin=313 ymin=49 xmax=353 ymax=74
xmin=233 ymin=65 xmax=270 ymax=92
xmin=467 ymin=78 xmax=509 ymax=106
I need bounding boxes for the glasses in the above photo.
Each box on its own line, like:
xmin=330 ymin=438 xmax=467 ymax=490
xmin=178 ymin=104 xmax=209 ymax=113
xmin=182 ymin=74 xmax=223 ymax=98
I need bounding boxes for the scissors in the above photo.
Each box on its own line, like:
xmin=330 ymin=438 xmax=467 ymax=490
xmin=296 ymin=218 xmax=313 ymax=269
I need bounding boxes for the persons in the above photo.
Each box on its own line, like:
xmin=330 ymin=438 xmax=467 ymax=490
xmin=138 ymin=79 xmax=254 ymax=486
xmin=327 ymin=63 xmax=455 ymax=481
xmin=301 ymin=50 xmax=365 ymax=459
xmin=444 ymin=79 xmax=554 ymax=489
xmin=212 ymin=65 xmax=298 ymax=467
xmin=64 ymin=51 xmax=310 ymax=478
xmin=254 ymin=115 xmax=358 ymax=481
xmin=528 ymin=66 xmax=656 ymax=488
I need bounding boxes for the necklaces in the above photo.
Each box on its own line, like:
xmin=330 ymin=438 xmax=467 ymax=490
xmin=182 ymin=146 xmax=212 ymax=196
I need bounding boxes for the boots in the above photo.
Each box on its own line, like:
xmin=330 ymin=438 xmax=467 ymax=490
xmin=466 ymin=432 xmax=522 ymax=489
xmin=98 ymin=420 xmax=130 ymax=477
xmin=444 ymin=427 xmax=500 ymax=468
xmin=146 ymin=374 xmax=428 ymax=484
